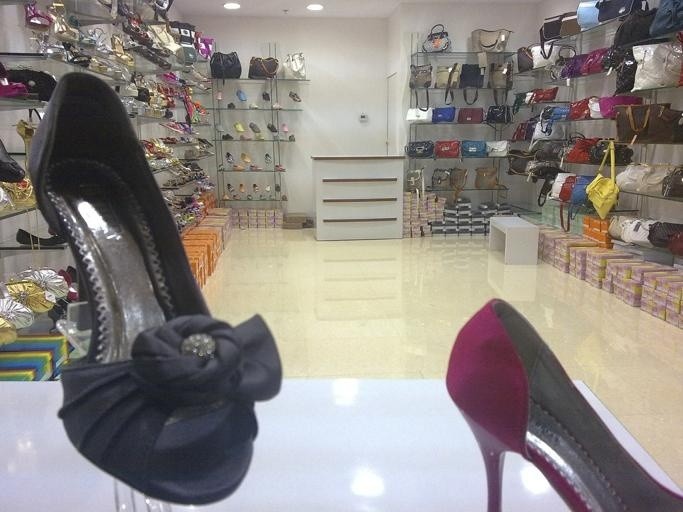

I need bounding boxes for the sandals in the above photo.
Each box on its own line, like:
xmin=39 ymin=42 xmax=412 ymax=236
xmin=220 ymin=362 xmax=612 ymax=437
xmin=117 ymin=70 xmax=215 ymax=231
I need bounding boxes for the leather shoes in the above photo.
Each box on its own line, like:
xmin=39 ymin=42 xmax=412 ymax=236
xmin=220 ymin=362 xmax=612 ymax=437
xmin=1 ymin=62 xmax=30 ymax=99
xmin=0 ymin=119 xmax=34 ymax=182
xmin=17 ymin=229 xmax=64 ymax=245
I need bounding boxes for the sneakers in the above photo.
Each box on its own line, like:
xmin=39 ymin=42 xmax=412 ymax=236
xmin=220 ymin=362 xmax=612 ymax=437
xmin=289 ymin=91 xmax=301 ymax=102
xmin=290 ymin=134 xmax=295 ymax=142
xmin=217 ymin=90 xmax=289 ymax=201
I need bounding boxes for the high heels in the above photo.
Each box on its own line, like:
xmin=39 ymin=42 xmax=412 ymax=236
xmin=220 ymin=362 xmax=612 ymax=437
xmin=447 ymin=296 xmax=683 ymax=511
xmin=33 ymin=72 xmax=282 ymax=505
xmin=24 ymin=1 xmax=133 ymax=79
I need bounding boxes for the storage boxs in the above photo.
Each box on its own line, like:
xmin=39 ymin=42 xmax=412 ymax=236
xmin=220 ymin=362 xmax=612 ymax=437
xmin=555 ymin=239 xmax=683 ymax=331
xmin=0 ymin=335 xmax=75 ymax=381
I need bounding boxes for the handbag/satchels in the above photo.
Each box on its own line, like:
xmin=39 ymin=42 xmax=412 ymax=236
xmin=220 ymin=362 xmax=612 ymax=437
xmin=127 ymin=0 xmax=214 ymax=64
xmin=610 ymin=216 xmax=683 ymax=256
xmin=514 ymin=1 xmax=683 ymax=219
xmin=282 ymin=52 xmax=306 ymax=79
xmin=248 ymin=57 xmax=279 ymax=79
xmin=406 ymin=23 xmax=513 ymax=191
xmin=211 ymin=51 xmax=241 ymax=80
